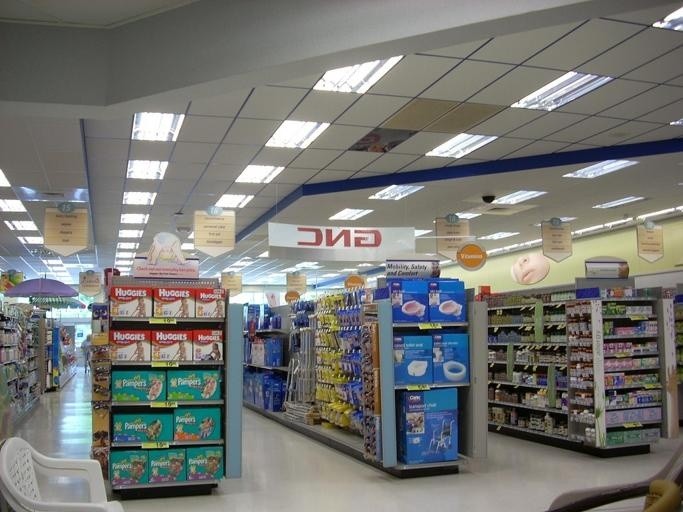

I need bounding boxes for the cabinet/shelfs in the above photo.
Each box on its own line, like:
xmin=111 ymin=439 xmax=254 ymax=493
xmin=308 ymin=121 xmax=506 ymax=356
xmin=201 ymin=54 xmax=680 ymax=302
xmin=244 ymin=280 xmax=683 ymax=480
xmin=2 ymin=303 xmax=45 ymax=432
xmin=91 ymin=282 xmax=242 ymax=498
xmin=46 ymin=324 xmax=76 ymax=392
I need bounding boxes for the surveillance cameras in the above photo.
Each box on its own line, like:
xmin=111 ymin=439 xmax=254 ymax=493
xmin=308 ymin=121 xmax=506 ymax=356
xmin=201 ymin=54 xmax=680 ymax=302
xmin=482 ymin=195 xmax=495 ymax=203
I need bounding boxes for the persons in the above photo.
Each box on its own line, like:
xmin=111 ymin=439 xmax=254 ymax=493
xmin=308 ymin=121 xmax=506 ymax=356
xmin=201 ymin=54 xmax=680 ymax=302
xmin=209 ymin=343 xmax=221 ymax=361
xmin=80 ymin=334 xmax=90 ymax=373
xmin=213 ymin=299 xmax=222 ymax=317
xmin=513 ymin=255 xmax=549 ymax=285
xmin=178 ymin=298 xmax=189 ymax=317
xmin=136 ymin=297 xmax=146 ymax=317
xmin=134 ymin=340 xmax=143 ymax=361
xmin=177 ymin=341 xmax=186 ymax=360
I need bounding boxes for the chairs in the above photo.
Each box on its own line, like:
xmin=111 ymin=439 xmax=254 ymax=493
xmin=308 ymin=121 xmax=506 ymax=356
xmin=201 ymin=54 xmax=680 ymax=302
xmin=1 ymin=440 xmax=126 ymax=512
